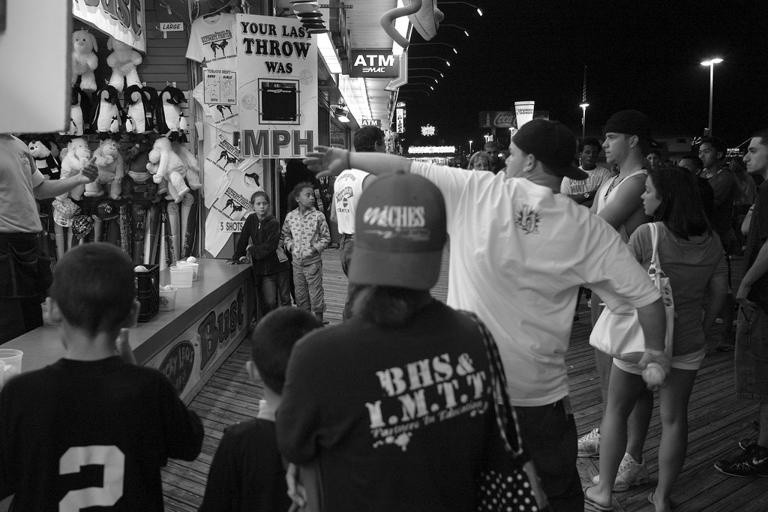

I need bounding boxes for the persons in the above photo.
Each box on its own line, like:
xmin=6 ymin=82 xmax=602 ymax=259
xmin=198 ymin=109 xmax=768 ymax=512
xmin=0 ymin=132 xmax=99 ymax=345
xmin=0 ymin=241 xmax=205 ymax=512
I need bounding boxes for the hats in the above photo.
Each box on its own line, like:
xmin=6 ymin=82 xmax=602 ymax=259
xmin=509 ymin=112 xmax=590 ymax=183
xmin=602 ymin=109 xmax=651 ymax=138
xmin=345 ymin=166 xmax=451 ymax=294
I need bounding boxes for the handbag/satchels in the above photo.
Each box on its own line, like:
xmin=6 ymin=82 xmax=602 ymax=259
xmin=585 ymin=273 xmax=676 ymax=369
xmin=472 ymin=467 xmax=540 ymax=512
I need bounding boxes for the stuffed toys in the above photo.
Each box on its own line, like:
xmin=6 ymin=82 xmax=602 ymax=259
xmin=55 ymin=86 xmax=91 ymax=141
xmin=70 ymin=28 xmax=98 ymax=92
xmin=124 ymin=84 xmax=153 ymax=140
xmin=88 ymin=86 xmax=124 ymax=140
xmin=140 ymin=86 xmax=159 ymax=134
xmin=106 ymin=38 xmax=145 ymax=91
xmin=28 ymin=131 xmax=204 ymax=228
xmin=155 ymin=84 xmax=191 ymax=144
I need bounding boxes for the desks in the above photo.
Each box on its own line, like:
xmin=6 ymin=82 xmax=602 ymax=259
xmin=0 ymin=258 xmax=257 ymax=511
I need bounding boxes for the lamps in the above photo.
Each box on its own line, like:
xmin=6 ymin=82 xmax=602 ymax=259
xmin=398 ymin=2 xmax=484 ymax=97
xmin=330 ymin=96 xmax=350 ymax=124
xmin=317 ymin=32 xmax=343 ymax=74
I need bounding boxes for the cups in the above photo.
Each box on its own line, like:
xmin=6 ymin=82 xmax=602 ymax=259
xmin=160 ymin=288 xmax=178 ymax=311
xmin=169 ymin=260 xmax=199 ymax=289
xmin=40 ymin=303 xmax=62 ymax=329
xmin=0 ymin=348 xmax=23 ymax=389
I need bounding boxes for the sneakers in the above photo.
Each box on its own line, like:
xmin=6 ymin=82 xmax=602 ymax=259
xmin=713 ymin=434 xmax=768 ymax=479
xmin=578 ymin=425 xmax=605 ymax=459
xmin=590 ymin=450 xmax=650 ymax=493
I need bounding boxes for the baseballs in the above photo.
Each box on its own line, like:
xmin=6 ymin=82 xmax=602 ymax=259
xmin=134 ymin=266 xmax=146 ymax=272
xmin=641 ymin=364 xmax=665 ymax=386
xmin=180 ymin=256 xmax=196 ymax=265
xmin=158 ymin=285 xmax=173 ymax=291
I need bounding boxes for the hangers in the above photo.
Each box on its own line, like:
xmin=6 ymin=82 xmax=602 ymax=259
xmin=203 ymin=0 xmax=221 ymax=19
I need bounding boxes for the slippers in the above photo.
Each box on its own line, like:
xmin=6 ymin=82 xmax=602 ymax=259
xmin=647 ymin=490 xmax=657 ymax=506
xmin=582 ymin=484 xmax=615 ymax=511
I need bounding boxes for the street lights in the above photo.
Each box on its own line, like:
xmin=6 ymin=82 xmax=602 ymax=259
xmin=469 ymin=140 xmax=473 ymax=154
xmin=508 ymin=127 xmax=514 ymax=143
xmin=698 ymin=56 xmax=724 ymax=140
xmin=579 ymin=102 xmax=589 ymax=135
xmin=483 ymin=136 xmax=488 ymax=143
